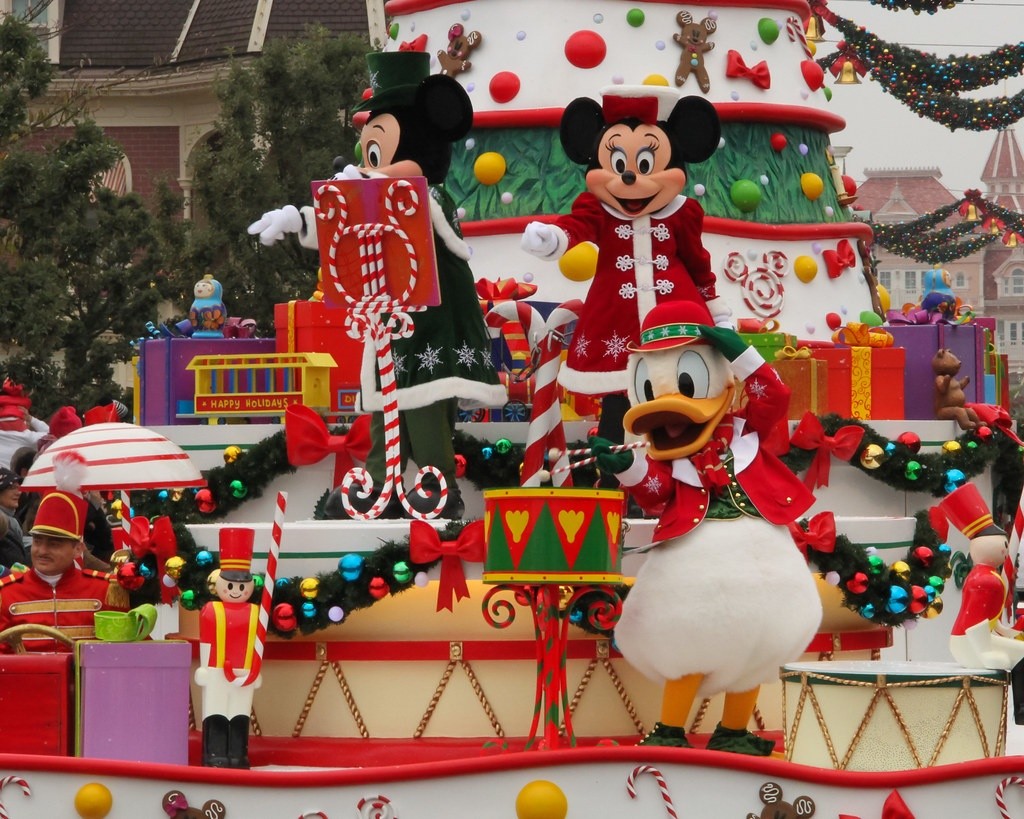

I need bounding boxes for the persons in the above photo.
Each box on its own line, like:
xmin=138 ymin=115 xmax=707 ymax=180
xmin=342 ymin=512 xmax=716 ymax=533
xmin=198 ymin=528 xmax=263 ymax=769
xmin=930 ymin=480 xmax=1024 ymax=729
xmin=0 ymin=378 xmax=152 ymax=655
xmin=188 ymin=276 xmax=226 ymax=339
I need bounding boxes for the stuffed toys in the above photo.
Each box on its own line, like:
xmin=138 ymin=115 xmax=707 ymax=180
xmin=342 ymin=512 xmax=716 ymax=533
xmin=588 ymin=300 xmax=823 ymax=755
xmin=521 ymin=95 xmax=733 ymax=522
xmin=251 ymin=50 xmax=510 ymax=521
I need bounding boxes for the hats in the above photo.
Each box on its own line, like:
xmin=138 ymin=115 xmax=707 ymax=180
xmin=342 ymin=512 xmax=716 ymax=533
xmin=84 ymin=397 xmax=130 ymax=427
xmin=28 ymin=451 xmax=89 ymax=543
xmin=0 ymin=467 xmax=24 ymax=491
xmin=49 ymin=406 xmax=82 ymax=438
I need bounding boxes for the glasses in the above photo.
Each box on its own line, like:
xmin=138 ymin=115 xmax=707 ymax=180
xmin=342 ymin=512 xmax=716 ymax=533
xmin=6 ymin=484 xmax=19 ymax=490
